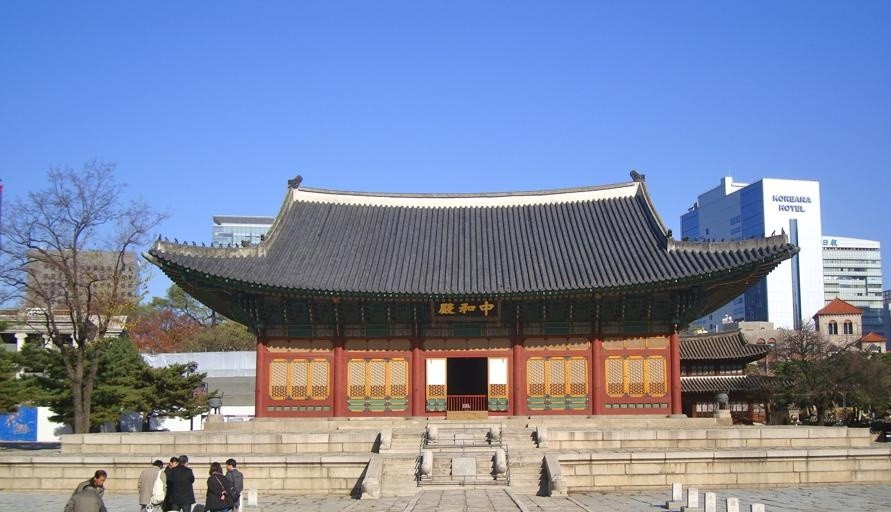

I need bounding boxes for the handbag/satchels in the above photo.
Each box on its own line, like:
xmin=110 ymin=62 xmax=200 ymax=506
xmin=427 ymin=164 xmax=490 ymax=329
xmin=229 ymin=486 xmax=240 ymax=500
xmin=214 ymin=493 xmax=232 ymax=509
xmin=151 ymin=470 xmax=165 ymax=505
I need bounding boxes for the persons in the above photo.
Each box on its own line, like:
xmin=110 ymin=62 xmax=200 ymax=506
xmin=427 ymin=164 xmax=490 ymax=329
xmin=63 ymin=485 xmax=107 ymax=512
xmin=137 ymin=455 xmax=244 ymax=511
xmin=75 ymin=470 xmax=107 ymax=499
